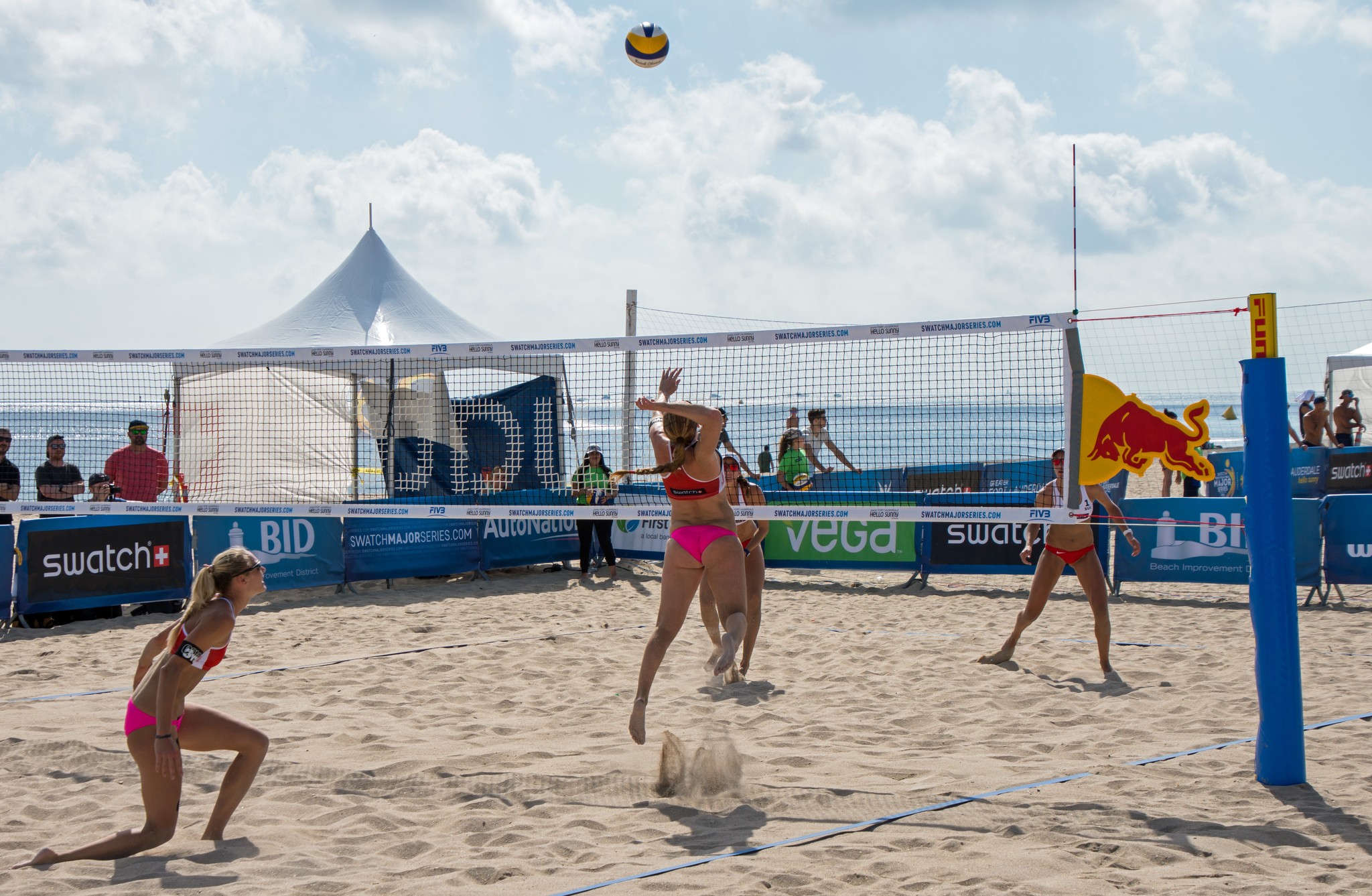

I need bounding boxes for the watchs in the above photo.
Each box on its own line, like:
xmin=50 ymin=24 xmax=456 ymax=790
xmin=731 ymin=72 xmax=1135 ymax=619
xmin=743 ymin=548 xmax=750 ymax=558
xmin=58 ymin=484 xmax=63 ymax=492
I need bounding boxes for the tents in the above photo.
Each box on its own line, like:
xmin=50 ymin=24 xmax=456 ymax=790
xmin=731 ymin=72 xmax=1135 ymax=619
xmin=175 ymin=226 xmax=567 ymax=591
xmin=1325 ymin=342 xmax=1372 ymax=448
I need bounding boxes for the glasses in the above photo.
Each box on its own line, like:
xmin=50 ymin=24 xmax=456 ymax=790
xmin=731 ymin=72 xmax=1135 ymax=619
xmin=723 ymin=415 xmax=727 ymax=419
xmin=49 ymin=444 xmax=66 ymax=449
xmin=0 ymin=436 xmax=12 ymax=443
xmin=723 ymin=463 xmax=738 ymax=471
xmin=131 ymin=429 xmax=147 ymax=435
xmin=1051 ymin=458 xmax=1064 ymax=465
xmin=228 ymin=561 xmax=261 ymax=580
xmin=791 ymin=411 xmax=796 ymax=413
xmin=823 ymin=417 xmax=826 ymax=420
xmin=93 ymin=476 xmax=110 ymax=481
xmin=1344 ymin=394 xmax=1354 ymax=397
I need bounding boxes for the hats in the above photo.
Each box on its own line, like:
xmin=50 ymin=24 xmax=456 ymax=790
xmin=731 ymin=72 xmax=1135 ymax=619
xmin=46 ymin=435 xmax=65 ymax=459
xmin=89 ymin=473 xmax=110 ymax=486
xmin=715 ymin=407 xmax=731 ymax=415
xmin=783 ymin=427 xmax=809 ymax=438
xmin=1339 ymin=389 xmax=1353 ymax=399
xmin=721 ymin=453 xmax=740 ymax=470
xmin=1313 ymin=395 xmax=1331 ymax=404
xmin=128 ymin=419 xmax=149 ymax=430
xmin=586 ymin=444 xmax=602 ymax=454
xmin=1052 ymin=447 xmax=1065 ymax=457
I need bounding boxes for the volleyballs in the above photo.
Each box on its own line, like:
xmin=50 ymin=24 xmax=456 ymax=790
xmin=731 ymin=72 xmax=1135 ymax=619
xmin=793 ymin=473 xmax=811 ymax=491
xmin=625 ymin=22 xmax=669 ymax=68
xmin=586 ymin=487 xmax=605 ymax=505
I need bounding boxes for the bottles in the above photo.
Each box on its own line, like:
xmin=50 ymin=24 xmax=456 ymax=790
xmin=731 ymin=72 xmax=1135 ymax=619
xmin=588 ymin=568 xmax=597 ymax=574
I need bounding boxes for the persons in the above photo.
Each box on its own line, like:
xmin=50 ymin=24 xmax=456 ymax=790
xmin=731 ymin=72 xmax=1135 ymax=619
xmin=607 ymin=366 xmax=749 ymax=744
xmin=11 ymin=549 xmax=271 ymax=870
xmin=697 ymin=452 xmax=769 ymax=679
xmin=0 ymin=428 xmax=20 ymax=526
xmin=35 ymin=436 xmax=85 ymax=517
xmin=1301 ymin=395 xmax=1344 ymax=449
xmin=104 ymin=419 xmax=169 ymax=502
xmin=84 ymin=474 xmax=132 ymax=502
xmin=1333 ymin=389 xmax=1366 ymax=447
xmin=568 ymin=403 xmax=1203 ymax=574
xmin=983 ymin=449 xmax=1141 ymax=686
xmin=1287 ymin=399 xmax=1308 ymax=451
xmin=1293 ymin=389 xmax=1325 ymax=447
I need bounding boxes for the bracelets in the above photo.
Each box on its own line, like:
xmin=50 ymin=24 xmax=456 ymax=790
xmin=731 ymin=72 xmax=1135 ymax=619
xmin=6 ymin=483 xmax=11 ymax=489
xmin=1299 ymin=444 xmax=1304 ymax=447
xmin=156 ymin=733 xmax=170 ymax=740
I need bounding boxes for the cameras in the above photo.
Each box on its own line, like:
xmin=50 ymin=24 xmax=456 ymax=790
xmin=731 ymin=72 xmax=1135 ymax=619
xmin=105 ymin=483 xmax=122 ymax=496
